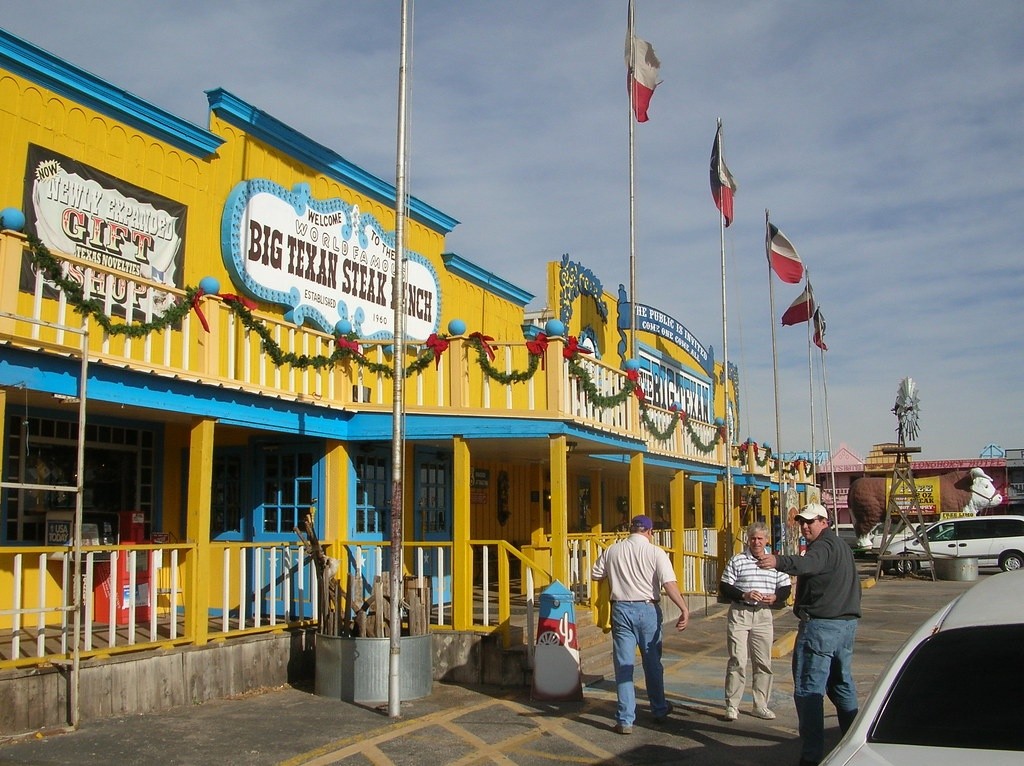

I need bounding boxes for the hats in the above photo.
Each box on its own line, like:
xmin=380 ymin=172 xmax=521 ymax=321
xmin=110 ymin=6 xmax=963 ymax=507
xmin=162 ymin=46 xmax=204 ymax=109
xmin=631 ymin=515 xmax=653 ymax=529
xmin=794 ymin=503 xmax=828 ymax=520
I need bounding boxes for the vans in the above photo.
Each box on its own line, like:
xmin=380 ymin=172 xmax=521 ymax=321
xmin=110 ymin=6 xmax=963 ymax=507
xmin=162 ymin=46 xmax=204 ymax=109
xmin=884 ymin=516 xmax=1024 ymax=576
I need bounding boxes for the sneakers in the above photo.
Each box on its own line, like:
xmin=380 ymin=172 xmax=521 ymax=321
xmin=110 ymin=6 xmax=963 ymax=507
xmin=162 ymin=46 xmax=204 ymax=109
xmin=615 ymin=724 xmax=632 ymax=733
xmin=724 ymin=709 xmax=739 ymax=720
xmin=656 ymin=701 xmax=673 ymax=721
xmin=751 ymin=708 xmax=776 ymax=719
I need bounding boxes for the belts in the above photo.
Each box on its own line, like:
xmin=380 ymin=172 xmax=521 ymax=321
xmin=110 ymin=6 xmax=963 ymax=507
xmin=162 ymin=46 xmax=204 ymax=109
xmin=614 ymin=600 xmax=656 ymax=603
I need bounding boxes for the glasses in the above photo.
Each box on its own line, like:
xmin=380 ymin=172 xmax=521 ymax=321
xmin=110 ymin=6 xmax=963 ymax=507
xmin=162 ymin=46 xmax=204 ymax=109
xmin=799 ymin=518 xmax=821 ymax=525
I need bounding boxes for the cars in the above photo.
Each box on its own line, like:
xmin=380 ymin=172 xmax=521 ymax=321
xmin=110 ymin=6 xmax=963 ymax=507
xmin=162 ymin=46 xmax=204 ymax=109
xmin=831 ymin=523 xmax=954 ymax=552
xmin=818 ymin=565 xmax=1024 ymax=766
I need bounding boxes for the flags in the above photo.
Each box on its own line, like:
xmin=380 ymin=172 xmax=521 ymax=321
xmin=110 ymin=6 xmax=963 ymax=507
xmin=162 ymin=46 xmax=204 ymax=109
xmin=812 ymin=310 xmax=829 ymax=354
xmin=779 ymin=279 xmax=814 ymax=328
xmin=625 ymin=4 xmax=662 ymax=123
xmin=765 ymin=219 xmax=803 ymax=286
xmin=709 ymin=129 xmax=738 ymax=228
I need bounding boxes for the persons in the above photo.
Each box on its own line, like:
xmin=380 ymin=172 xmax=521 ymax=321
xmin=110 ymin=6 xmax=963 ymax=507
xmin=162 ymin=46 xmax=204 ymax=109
xmin=754 ymin=501 xmax=861 ymax=766
xmin=716 ymin=528 xmax=792 ymax=720
xmin=591 ymin=513 xmax=689 ymax=733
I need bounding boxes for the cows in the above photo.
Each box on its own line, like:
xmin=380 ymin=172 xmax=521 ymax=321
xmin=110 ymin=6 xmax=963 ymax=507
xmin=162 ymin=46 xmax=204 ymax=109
xmin=847 ymin=467 xmax=1003 ymax=555
